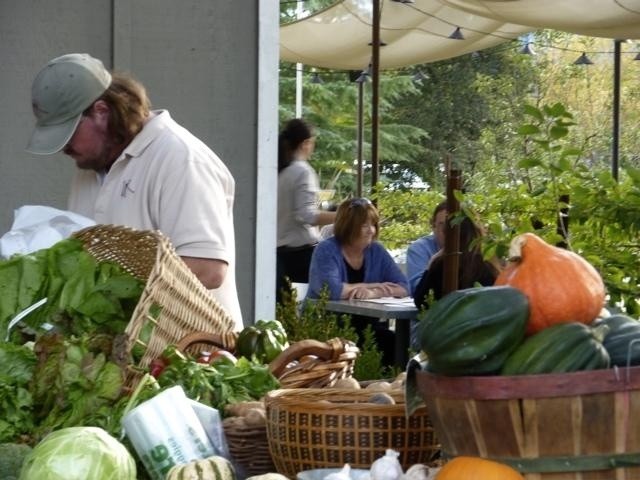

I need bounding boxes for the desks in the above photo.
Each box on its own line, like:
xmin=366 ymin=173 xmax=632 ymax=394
xmin=314 ymin=295 xmax=421 ymax=371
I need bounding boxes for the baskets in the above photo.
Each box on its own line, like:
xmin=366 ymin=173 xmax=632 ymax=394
xmin=70 ymin=224 xmax=441 ymax=480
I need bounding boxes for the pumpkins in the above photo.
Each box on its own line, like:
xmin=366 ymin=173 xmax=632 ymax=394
xmin=164 ymin=455 xmax=238 ymax=480
xmin=244 ymin=472 xmax=292 ymax=480
xmin=410 ymin=232 xmax=639 ymax=379
xmin=435 ymin=455 xmax=525 ymax=480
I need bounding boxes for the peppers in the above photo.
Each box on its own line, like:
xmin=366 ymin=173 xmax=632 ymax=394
xmin=126 ymin=318 xmax=293 ymax=419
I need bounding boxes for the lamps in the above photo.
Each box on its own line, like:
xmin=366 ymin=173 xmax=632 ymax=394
xmin=519 ymin=43 xmax=536 ymax=56
xmin=633 ymin=53 xmax=639 ymax=61
xmin=355 ymin=72 xmax=373 ymax=84
xmin=448 ymin=28 xmax=465 ymax=40
xmin=571 ymin=52 xmax=595 ymax=66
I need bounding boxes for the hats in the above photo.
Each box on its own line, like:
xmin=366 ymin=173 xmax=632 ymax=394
xmin=25 ymin=53 xmax=112 ymax=156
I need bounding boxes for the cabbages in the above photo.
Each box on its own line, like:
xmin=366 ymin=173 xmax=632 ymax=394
xmin=17 ymin=425 xmax=137 ymax=480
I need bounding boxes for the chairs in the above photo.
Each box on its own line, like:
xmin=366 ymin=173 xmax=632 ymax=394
xmin=290 ymin=282 xmax=309 ymax=315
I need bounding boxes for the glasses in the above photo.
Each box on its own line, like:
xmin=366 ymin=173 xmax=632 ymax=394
xmin=351 ymin=197 xmax=372 ymax=207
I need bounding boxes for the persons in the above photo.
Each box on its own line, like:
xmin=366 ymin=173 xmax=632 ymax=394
xmin=25 ymin=53 xmax=244 ymax=339
xmin=278 ymin=118 xmax=340 ymax=281
xmin=414 ymin=214 xmax=500 ymax=310
xmin=300 ymin=196 xmax=413 ymax=372
xmin=406 ymin=201 xmax=450 ymax=354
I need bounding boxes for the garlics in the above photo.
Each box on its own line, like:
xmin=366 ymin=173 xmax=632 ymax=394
xmin=320 ymin=447 xmax=439 ymax=479
